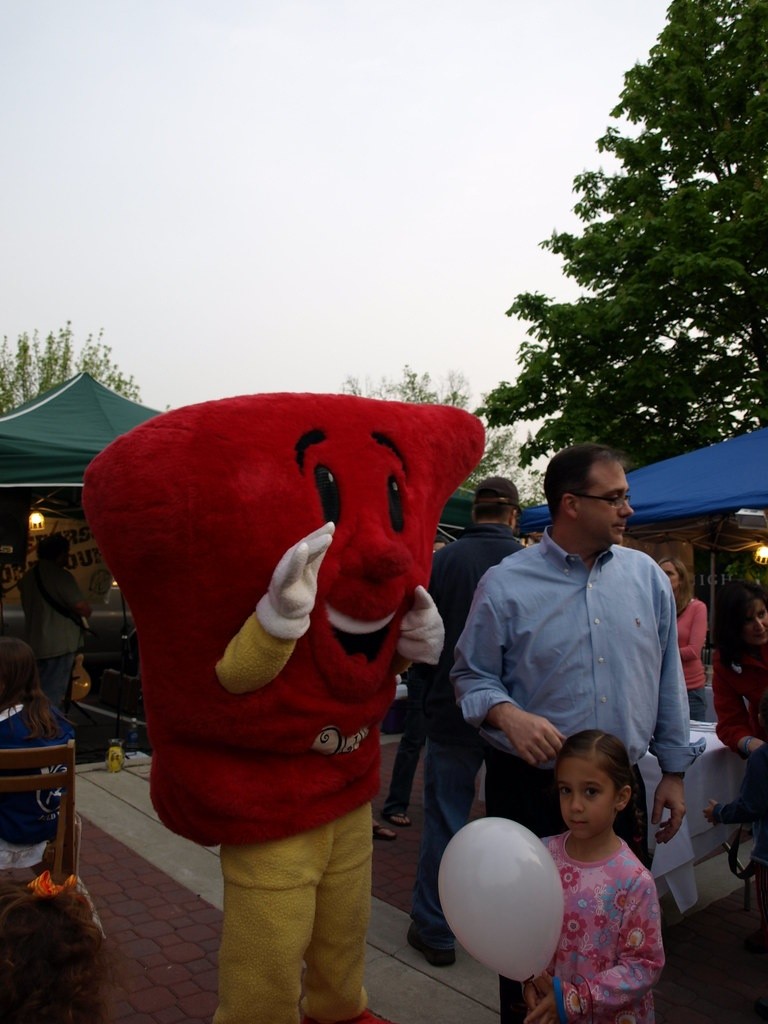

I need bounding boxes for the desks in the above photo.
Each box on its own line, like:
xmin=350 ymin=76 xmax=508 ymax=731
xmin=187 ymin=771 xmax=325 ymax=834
xmin=639 ymin=715 xmax=754 ymax=912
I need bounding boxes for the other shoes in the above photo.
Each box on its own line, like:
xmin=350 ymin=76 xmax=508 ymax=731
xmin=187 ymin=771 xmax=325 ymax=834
xmin=755 ymin=997 xmax=768 ymax=1022
xmin=744 ymin=927 xmax=768 ymax=954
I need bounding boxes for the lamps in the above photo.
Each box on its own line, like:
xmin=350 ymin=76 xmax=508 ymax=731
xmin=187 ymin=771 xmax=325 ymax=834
xmin=27 ymin=505 xmax=47 ymax=531
xmin=734 ymin=507 xmax=768 ymax=530
xmin=752 ymin=544 xmax=768 ymax=565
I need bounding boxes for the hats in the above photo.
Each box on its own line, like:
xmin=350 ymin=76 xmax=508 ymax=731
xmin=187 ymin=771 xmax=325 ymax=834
xmin=474 ymin=475 xmax=524 ymax=517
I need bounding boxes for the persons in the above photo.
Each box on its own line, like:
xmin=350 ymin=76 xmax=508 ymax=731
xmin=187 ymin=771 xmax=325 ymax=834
xmin=703 ymin=579 xmax=768 ymax=1024
xmin=658 ymin=556 xmax=708 ymax=722
xmin=372 ymin=477 xmax=526 ymax=969
xmin=0 ymin=639 xmax=75 ymax=869
xmin=0 ymin=870 xmax=113 ymax=1024
xmin=522 ymin=728 xmax=665 ymax=1024
xmin=19 ymin=534 xmax=93 ymax=756
xmin=448 ymin=443 xmax=706 ymax=1024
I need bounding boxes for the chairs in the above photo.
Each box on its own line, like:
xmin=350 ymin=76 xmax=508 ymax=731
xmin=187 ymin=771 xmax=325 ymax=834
xmin=0 ymin=738 xmax=89 ymax=900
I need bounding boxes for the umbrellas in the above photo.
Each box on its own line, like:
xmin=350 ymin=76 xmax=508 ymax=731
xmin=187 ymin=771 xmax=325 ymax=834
xmin=515 ymin=427 xmax=768 ymax=647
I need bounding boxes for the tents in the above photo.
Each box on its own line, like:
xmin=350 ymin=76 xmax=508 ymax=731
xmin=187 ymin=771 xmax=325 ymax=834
xmin=0 ymin=372 xmax=475 ymax=542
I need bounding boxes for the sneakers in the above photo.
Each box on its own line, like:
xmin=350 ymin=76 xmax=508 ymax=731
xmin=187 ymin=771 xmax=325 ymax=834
xmin=407 ymin=921 xmax=456 ymax=967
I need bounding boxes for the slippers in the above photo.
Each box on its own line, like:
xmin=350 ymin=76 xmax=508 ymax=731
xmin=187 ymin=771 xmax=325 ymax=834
xmin=380 ymin=810 xmax=412 ymax=827
xmin=373 ymin=825 xmax=397 ymax=840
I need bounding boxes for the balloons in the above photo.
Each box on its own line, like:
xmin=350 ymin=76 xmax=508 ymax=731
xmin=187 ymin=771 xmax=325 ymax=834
xmin=437 ymin=817 xmax=565 ymax=1000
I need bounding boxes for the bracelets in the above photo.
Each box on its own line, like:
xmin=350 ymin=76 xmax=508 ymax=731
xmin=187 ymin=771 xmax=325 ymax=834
xmin=741 ymin=735 xmax=754 ymax=754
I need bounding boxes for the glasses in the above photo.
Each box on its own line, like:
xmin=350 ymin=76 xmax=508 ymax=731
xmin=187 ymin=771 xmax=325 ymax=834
xmin=576 ymin=494 xmax=632 ymax=509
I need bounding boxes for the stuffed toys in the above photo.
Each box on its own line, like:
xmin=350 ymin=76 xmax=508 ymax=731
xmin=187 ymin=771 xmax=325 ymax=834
xmin=82 ymin=392 xmax=486 ymax=1024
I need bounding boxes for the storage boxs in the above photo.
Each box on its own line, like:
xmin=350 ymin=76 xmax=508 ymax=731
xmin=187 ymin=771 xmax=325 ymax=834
xmin=99 ymin=667 xmax=137 ymax=713
